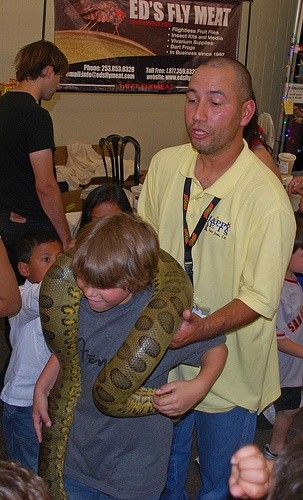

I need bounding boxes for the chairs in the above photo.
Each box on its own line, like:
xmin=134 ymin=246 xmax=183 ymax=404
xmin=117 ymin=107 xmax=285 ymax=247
xmin=99 ymin=134 xmax=140 ymax=187
xmin=257 ymin=111 xmax=274 ymax=155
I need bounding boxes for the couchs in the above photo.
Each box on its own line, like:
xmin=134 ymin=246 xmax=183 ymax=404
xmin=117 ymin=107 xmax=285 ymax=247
xmin=53 ymin=142 xmax=148 ymax=213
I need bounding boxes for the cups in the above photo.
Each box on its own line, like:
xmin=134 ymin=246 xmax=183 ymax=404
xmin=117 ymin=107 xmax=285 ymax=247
xmin=131 ymin=186 xmax=143 ymax=208
xmin=279 ymin=153 xmax=296 ymax=176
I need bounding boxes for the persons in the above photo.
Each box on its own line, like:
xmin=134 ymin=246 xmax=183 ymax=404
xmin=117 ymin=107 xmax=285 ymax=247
xmin=288 ymin=176 xmax=303 ymax=212
xmin=32 ymin=213 xmax=229 ymax=500
xmin=0 ymin=228 xmax=76 ymax=475
xmin=228 ymin=434 xmax=303 ymax=500
xmin=0 ymin=235 xmax=22 ymax=318
xmin=68 ymin=185 xmax=134 ymax=250
xmin=137 ymin=56 xmax=296 ymax=500
xmin=264 ymin=211 xmax=303 ymax=460
xmin=194 ymin=89 xmax=283 ymax=466
xmin=0 ymin=461 xmax=51 ymax=500
xmin=0 ymin=41 xmax=73 ymax=387
